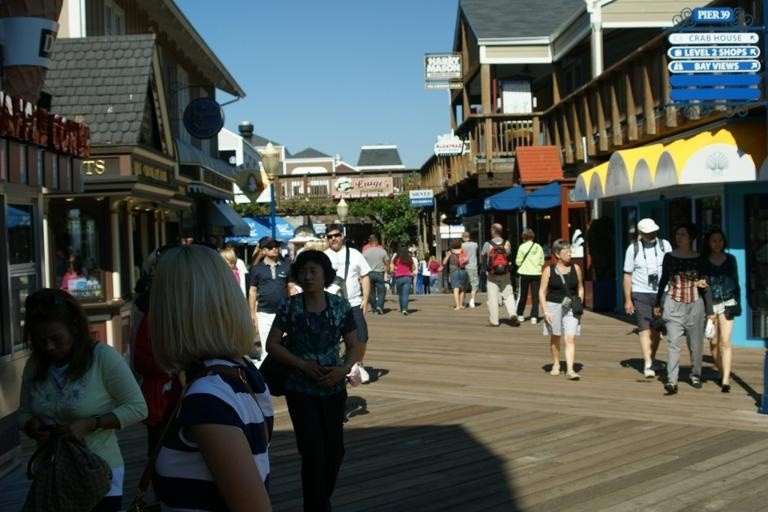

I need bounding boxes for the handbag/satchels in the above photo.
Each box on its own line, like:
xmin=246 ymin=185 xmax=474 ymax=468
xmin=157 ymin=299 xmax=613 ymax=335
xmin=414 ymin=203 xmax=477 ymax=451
xmin=512 ymin=241 xmax=537 ymax=279
xmin=554 ymin=268 xmax=584 ymax=317
xmin=332 ymin=244 xmax=350 ymax=300
xmin=124 ymin=364 xmax=270 ymax=512
xmin=257 ymin=294 xmax=305 ymax=396
xmin=712 ymin=261 xmax=742 ymax=320
xmin=458 ymin=249 xmax=469 ymax=267
xmin=25 ymin=429 xmax=114 ymax=508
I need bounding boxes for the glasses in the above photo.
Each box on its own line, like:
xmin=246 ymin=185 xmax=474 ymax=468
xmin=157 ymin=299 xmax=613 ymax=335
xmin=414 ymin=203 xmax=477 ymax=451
xmin=325 ymin=232 xmax=344 ymax=240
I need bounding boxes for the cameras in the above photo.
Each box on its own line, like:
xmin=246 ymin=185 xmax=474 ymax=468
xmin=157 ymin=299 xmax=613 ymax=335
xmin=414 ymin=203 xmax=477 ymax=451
xmin=648 ymin=273 xmax=659 ymax=285
xmin=560 ymin=296 xmax=572 ymax=309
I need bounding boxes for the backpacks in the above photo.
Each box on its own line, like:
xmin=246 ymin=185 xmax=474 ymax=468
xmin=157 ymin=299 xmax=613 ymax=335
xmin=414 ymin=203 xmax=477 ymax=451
xmin=487 ymin=239 xmax=510 ymax=275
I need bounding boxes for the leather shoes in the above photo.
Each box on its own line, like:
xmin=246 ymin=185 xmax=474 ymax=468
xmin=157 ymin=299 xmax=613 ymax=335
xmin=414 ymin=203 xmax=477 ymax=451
xmin=664 ymin=383 xmax=678 ymax=393
xmin=722 ymin=381 xmax=731 ymax=392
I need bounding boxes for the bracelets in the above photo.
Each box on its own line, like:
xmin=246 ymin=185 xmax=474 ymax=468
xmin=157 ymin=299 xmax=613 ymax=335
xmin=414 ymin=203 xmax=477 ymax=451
xmin=343 ymin=365 xmax=351 ymax=374
xmin=93 ymin=415 xmax=101 ymax=429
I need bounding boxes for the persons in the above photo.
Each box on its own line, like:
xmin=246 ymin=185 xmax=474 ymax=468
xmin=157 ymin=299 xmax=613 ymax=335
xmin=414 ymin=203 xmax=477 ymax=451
xmin=265 ymin=250 xmax=361 ymax=512
xmin=362 ymin=237 xmax=390 ymax=314
xmin=322 ymin=224 xmax=373 ymax=383
xmin=419 ymin=257 xmax=431 ymax=294
xmin=249 ymin=238 xmax=292 ymax=356
xmin=461 ymin=231 xmax=479 ymax=308
xmin=516 ymin=229 xmax=545 ymax=325
xmin=149 ymin=245 xmax=274 ymax=512
xmin=482 ymin=223 xmax=521 ymax=327
xmin=391 ymin=243 xmax=414 ymax=314
xmin=443 ymin=240 xmax=468 ymax=310
xmin=219 ymin=250 xmax=239 ymax=288
xmin=363 ymin=234 xmax=384 ymax=253
xmin=223 ymin=242 xmax=249 ymax=304
xmin=428 ymin=256 xmax=440 ymax=294
xmin=654 ymin=225 xmax=716 ymax=391
xmin=539 ymin=239 xmax=584 ymax=380
xmin=135 ymin=275 xmax=183 ymax=457
xmin=19 ymin=290 xmax=147 ymax=512
xmin=624 ymin=218 xmax=672 ymax=377
xmin=696 ymin=230 xmax=741 ymax=390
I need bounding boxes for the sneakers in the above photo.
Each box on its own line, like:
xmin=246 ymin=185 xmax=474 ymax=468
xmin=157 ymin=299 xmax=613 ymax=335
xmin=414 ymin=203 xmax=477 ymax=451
xmin=643 ymin=367 xmax=654 ymax=377
xmin=690 ymin=375 xmax=701 ymax=388
xmin=376 ymin=306 xmax=383 ymax=316
xmin=510 ymin=314 xmax=521 ymax=327
xmin=567 ymin=370 xmax=580 ymax=378
xmin=402 ymin=310 xmax=409 ymax=316
xmin=549 ymin=367 xmax=561 ymax=375
xmin=357 ymin=363 xmax=371 ymax=386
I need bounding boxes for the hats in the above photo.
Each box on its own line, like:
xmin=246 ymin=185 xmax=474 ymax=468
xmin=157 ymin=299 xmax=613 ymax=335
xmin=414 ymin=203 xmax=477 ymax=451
xmin=639 ymin=219 xmax=659 ymax=234
xmin=259 ymin=237 xmax=281 ymax=248
